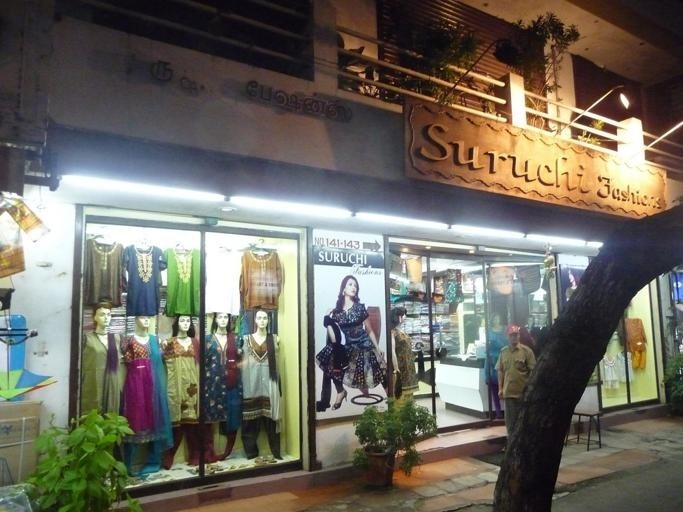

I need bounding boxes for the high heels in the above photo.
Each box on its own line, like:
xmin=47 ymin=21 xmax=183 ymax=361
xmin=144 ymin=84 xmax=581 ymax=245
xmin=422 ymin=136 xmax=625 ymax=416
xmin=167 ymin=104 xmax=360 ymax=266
xmin=331 ymin=390 xmax=348 ymax=409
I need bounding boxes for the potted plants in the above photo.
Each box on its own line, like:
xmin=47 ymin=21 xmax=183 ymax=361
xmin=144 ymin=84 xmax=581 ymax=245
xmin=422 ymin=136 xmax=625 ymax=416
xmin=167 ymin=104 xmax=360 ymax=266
xmin=349 ymin=389 xmax=441 ymax=489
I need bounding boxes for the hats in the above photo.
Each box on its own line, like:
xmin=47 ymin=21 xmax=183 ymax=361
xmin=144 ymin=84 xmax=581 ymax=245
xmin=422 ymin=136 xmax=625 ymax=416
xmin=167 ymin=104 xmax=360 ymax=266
xmin=507 ymin=324 xmax=520 ymax=335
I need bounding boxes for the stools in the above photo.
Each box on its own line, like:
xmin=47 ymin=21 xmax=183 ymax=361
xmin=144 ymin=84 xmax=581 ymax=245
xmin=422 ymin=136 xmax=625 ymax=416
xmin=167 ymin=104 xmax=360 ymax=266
xmin=564 ymin=407 xmax=604 ymax=452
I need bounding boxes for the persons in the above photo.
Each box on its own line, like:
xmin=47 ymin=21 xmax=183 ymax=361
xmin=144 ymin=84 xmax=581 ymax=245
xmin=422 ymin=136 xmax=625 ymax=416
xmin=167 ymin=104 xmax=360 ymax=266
xmin=391 ymin=306 xmax=420 ymax=403
xmin=159 ymin=312 xmax=203 ymax=468
xmin=564 ymin=268 xmax=581 ymax=300
xmin=242 ymin=309 xmax=285 ymax=459
xmin=205 ymin=309 xmax=243 ymax=463
xmin=315 ymin=275 xmax=391 ymax=410
xmin=494 ymin=324 xmax=536 ymax=453
xmin=120 ymin=310 xmax=170 ymax=474
xmin=81 ymin=301 xmax=128 ymax=440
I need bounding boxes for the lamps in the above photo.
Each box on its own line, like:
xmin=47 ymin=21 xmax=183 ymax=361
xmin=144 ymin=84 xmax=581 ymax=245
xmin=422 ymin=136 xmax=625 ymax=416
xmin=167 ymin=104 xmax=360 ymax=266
xmin=437 ymin=37 xmax=522 ymax=107
xmin=556 ymin=82 xmax=631 ymax=138
xmin=388 ymin=255 xmax=408 ymax=284
